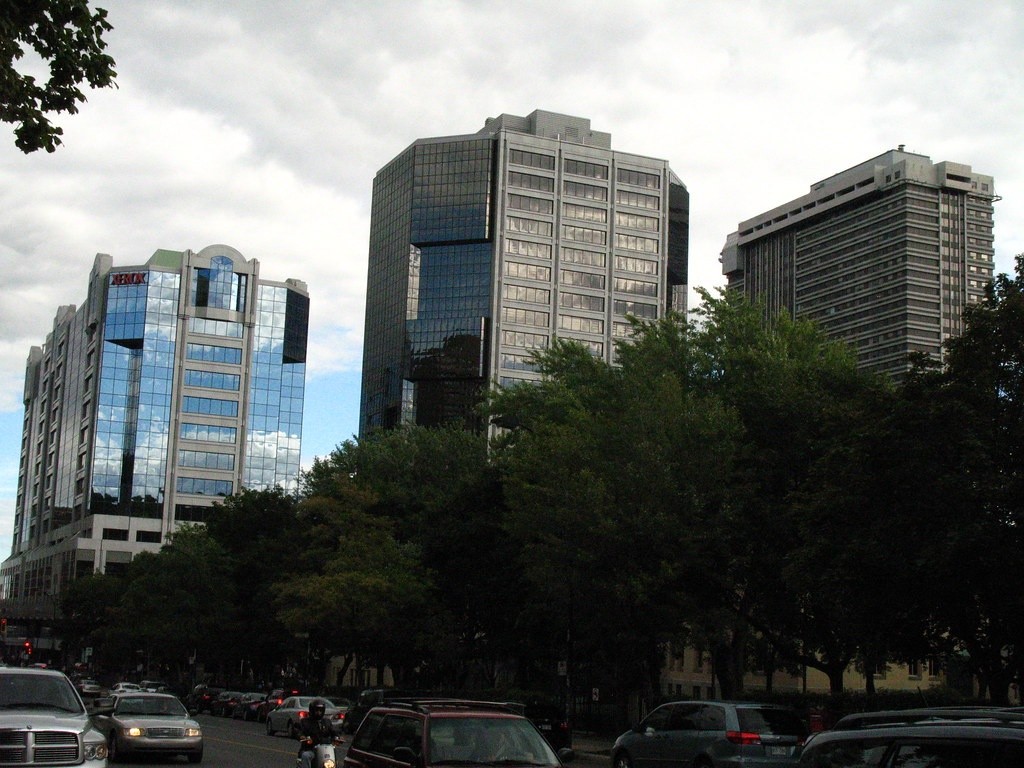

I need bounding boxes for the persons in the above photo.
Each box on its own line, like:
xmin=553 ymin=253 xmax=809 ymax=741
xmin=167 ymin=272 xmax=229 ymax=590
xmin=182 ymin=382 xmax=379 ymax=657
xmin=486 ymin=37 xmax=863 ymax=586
xmin=296 ymin=700 xmax=346 ymax=768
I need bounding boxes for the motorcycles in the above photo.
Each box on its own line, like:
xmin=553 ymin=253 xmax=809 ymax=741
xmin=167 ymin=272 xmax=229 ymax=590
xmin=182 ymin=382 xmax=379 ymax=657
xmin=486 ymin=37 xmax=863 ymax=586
xmin=295 ymin=735 xmax=348 ymax=768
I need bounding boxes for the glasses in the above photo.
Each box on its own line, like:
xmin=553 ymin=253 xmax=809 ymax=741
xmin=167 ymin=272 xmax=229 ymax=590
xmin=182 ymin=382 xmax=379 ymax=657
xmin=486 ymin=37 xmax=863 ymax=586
xmin=314 ymin=707 xmax=325 ymax=712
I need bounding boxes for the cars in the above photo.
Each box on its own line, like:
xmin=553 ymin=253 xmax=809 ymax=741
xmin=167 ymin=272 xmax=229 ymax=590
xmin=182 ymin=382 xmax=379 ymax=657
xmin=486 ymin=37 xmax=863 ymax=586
xmin=611 ymin=699 xmax=811 ymax=768
xmin=788 ymin=686 xmax=1024 ymax=768
xmin=66 ymin=675 xmax=415 ymax=740
xmin=343 ymin=696 xmax=577 ymax=768
xmin=0 ymin=666 xmax=109 ymax=768
xmin=85 ymin=691 xmax=204 ymax=765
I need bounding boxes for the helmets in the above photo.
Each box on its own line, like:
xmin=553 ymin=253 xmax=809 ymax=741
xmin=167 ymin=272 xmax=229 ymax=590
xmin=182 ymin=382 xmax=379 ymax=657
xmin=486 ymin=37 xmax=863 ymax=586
xmin=309 ymin=699 xmax=326 ymax=719
